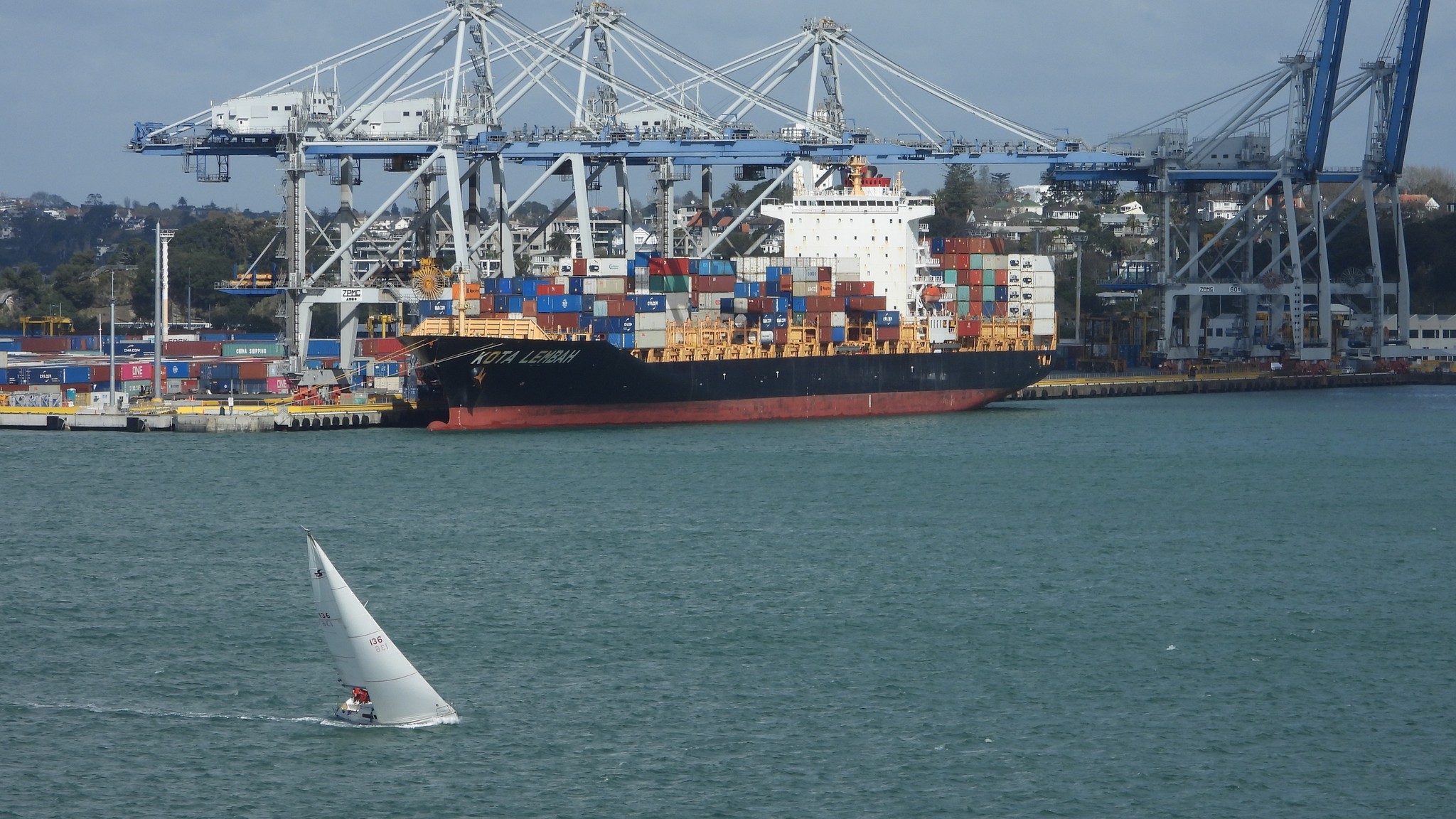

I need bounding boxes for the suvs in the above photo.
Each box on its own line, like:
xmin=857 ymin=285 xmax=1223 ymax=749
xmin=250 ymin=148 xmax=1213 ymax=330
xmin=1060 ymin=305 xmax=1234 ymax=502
xmin=1211 ymin=358 xmax=1227 ymax=364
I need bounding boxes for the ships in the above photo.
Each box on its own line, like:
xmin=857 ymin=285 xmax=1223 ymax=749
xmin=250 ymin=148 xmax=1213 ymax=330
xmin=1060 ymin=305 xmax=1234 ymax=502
xmin=390 ymin=154 xmax=1083 ymax=433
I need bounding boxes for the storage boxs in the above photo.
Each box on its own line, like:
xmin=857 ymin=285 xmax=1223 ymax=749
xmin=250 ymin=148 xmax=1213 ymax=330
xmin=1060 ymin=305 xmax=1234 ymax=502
xmin=0 ymin=330 xmax=417 ymax=409
xmin=419 ymin=238 xmax=1055 ymax=348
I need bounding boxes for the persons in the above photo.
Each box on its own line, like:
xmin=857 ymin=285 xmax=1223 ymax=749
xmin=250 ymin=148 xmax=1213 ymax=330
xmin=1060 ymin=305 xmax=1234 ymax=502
xmin=353 ymin=688 xmax=371 ymax=703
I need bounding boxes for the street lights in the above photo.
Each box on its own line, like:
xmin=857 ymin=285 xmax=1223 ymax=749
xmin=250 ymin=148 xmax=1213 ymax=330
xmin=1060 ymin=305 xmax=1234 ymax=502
xmin=109 ymin=299 xmax=115 ymax=406
xmin=151 ymin=228 xmax=178 ymax=343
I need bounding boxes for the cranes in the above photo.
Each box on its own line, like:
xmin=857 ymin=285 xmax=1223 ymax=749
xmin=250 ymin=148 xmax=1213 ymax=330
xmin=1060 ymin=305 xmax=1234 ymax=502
xmin=1053 ymin=0 xmax=1432 ymax=363
xmin=124 ymin=2 xmax=1074 ymax=368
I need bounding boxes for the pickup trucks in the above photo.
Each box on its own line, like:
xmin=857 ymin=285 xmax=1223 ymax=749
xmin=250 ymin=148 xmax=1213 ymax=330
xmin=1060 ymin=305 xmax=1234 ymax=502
xmin=1342 ymin=367 xmax=1355 ymax=374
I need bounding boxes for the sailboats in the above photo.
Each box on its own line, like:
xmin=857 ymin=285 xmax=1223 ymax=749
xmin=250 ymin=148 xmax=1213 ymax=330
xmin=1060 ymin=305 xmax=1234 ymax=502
xmin=301 ymin=527 xmax=460 ymax=729
